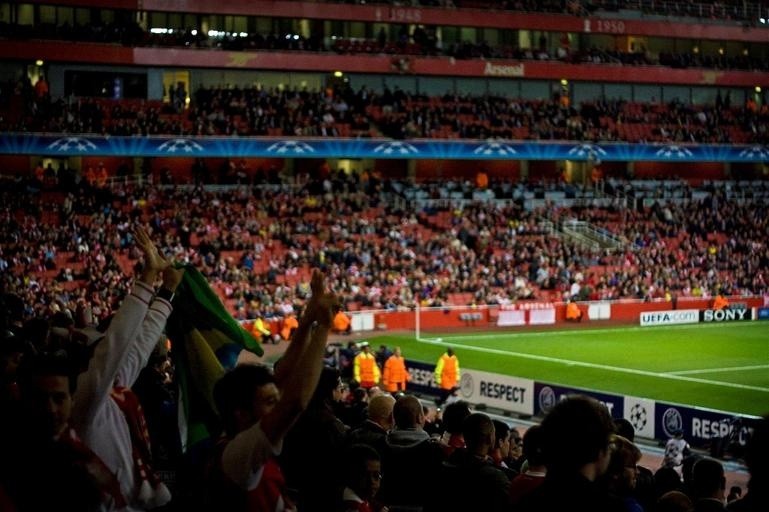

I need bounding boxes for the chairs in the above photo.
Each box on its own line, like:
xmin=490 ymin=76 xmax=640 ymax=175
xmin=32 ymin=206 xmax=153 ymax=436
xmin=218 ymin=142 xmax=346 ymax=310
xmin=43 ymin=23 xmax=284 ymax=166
xmin=2 ymin=178 xmax=768 ymax=314
xmin=3 ymin=93 xmax=768 ymax=142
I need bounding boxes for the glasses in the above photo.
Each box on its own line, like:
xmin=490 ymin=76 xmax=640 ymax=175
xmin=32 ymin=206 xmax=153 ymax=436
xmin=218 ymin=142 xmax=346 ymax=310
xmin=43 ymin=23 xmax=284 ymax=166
xmin=623 ymin=462 xmax=638 ymax=469
xmin=607 ymin=439 xmax=624 ymax=452
